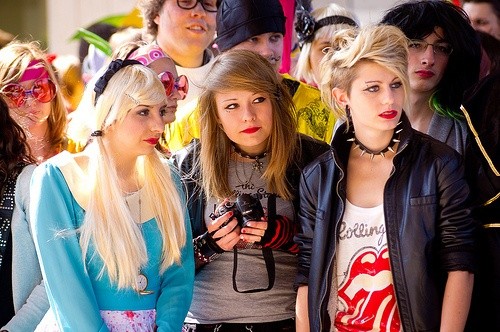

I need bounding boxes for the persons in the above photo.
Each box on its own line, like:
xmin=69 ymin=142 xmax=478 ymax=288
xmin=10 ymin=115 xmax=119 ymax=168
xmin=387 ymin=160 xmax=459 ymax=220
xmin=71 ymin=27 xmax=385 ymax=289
xmin=0 ymin=96 xmax=31 ymax=320
xmin=173 ymin=5 xmax=335 ymax=157
xmin=63 ymin=0 xmax=216 ymax=148
xmin=303 ymin=14 xmax=364 ymax=104
xmin=0 ymin=36 xmax=65 ymax=165
xmin=465 ymin=21 xmax=500 ymax=143
xmin=457 ymin=0 xmax=500 ymax=42
xmin=289 ymin=24 xmax=483 ymax=332
xmin=31 ymin=58 xmax=197 ymax=332
xmin=174 ymin=48 xmax=337 ymax=332
xmin=51 ymin=55 xmax=83 ymax=112
xmin=378 ymin=1 xmax=476 ymax=175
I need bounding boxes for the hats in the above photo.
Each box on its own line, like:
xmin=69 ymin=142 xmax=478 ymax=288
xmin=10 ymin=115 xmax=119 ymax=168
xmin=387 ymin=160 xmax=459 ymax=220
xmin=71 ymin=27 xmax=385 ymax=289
xmin=215 ymin=0 xmax=288 ymax=53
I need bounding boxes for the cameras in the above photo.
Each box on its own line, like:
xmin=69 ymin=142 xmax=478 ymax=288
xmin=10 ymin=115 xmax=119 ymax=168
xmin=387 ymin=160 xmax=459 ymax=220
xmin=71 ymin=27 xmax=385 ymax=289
xmin=216 ymin=193 xmax=266 ymax=235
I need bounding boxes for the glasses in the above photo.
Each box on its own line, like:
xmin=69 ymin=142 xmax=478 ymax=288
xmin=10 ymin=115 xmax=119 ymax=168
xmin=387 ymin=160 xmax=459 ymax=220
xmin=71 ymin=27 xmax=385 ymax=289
xmin=0 ymin=80 xmax=55 ymax=105
xmin=176 ymin=0 xmax=219 ymax=12
xmin=157 ymin=71 xmax=189 ymax=100
xmin=405 ymin=35 xmax=456 ymax=54
